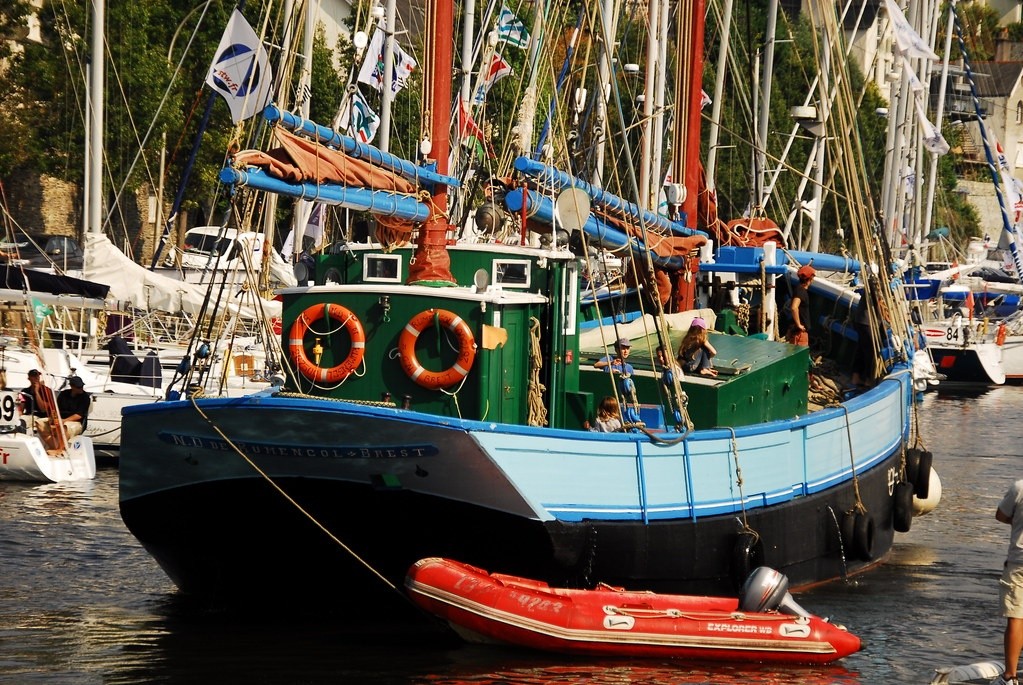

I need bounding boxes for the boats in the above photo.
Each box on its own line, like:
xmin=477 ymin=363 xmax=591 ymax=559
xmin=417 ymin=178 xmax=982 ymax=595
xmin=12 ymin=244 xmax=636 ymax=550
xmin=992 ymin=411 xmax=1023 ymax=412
xmin=404 ymin=553 xmax=862 ymax=666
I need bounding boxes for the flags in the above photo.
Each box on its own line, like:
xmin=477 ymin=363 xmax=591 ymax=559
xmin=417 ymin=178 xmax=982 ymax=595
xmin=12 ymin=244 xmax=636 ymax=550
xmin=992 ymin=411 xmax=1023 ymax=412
xmin=206 ymin=8 xmax=274 ymax=123
xmin=886 ymin=0 xmax=949 ymax=203
xmin=474 ymin=50 xmax=514 ymax=106
xmin=358 ymin=20 xmax=416 ymax=102
xmin=990 ymin=131 xmax=1023 ymax=245
xmin=340 ymin=87 xmax=381 ymax=144
xmin=497 ymin=5 xmax=531 ymax=50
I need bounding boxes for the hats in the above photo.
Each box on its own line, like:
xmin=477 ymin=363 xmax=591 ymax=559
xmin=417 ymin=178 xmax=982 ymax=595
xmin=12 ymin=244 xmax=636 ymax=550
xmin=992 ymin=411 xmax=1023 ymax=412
xmin=69 ymin=376 xmax=85 ymax=387
xmin=614 ymin=338 xmax=632 ymax=347
xmin=797 ymin=266 xmax=815 ymax=280
xmin=691 ymin=317 xmax=706 ymax=330
xmin=28 ymin=369 xmax=41 ymax=377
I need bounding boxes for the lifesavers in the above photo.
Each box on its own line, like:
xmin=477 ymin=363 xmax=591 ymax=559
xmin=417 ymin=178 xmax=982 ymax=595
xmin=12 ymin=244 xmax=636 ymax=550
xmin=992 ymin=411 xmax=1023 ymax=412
xmin=911 ymin=306 xmax=924 ymax=324
xmin=907 ymin=447 xmax=933 ymax=500
xmin=893 ymin=482 xmax=915 ymax=533
xmin=398 ymin=307 xmax=476 ymax=391
xmin=734 ymin=533 xmax=766 ymax=589
xmin=997 ymin=324 xmax=1006 ymax=345
xmin=843 ymin=511 xmax=877 ymax=562
xmin=289 ymin=302 xmax=366 ymax=383
xmin=952 ymin=262 xmax=958 ymax=279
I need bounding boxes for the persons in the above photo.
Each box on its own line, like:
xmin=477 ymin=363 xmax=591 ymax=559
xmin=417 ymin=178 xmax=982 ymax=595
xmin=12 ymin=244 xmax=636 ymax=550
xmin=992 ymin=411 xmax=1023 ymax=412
xmin=35 ymin=376 xmax=91 ymax=455
xmin=783 ymin=265 xmax=816 ymax=346
xmin=908 ymin=243 xmax=921 ymax=280
xmin=18 ymin=369 xmax=53 ymax=428
xmin=989 ymin=479 xmax=1023 ymax=685
xmin=584 ymin=397 xmax=621 ymax=433
xmin=593 ymin=338 xmax=633 ymax=379
xmin=0 ymin=368 xmax=25 ymax=416
xmin=657 ymin=346 xmax=685 ymax=388
xmin=677 ymin=317 xmax=718 ymax=378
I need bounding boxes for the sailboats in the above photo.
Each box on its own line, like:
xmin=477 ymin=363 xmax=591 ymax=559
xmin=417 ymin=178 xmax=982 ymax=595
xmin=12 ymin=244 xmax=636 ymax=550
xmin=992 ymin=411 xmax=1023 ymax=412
xmin=1 ymin=0 xmax=1023 ymax=483
xmin=118 ymin=1 xmax=944 ymax=646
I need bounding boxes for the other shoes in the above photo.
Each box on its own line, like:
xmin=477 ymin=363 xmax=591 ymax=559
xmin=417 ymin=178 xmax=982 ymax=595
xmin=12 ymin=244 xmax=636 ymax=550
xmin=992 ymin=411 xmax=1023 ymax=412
xmin=989 ymin=674 xmax=1018 ymax=685
